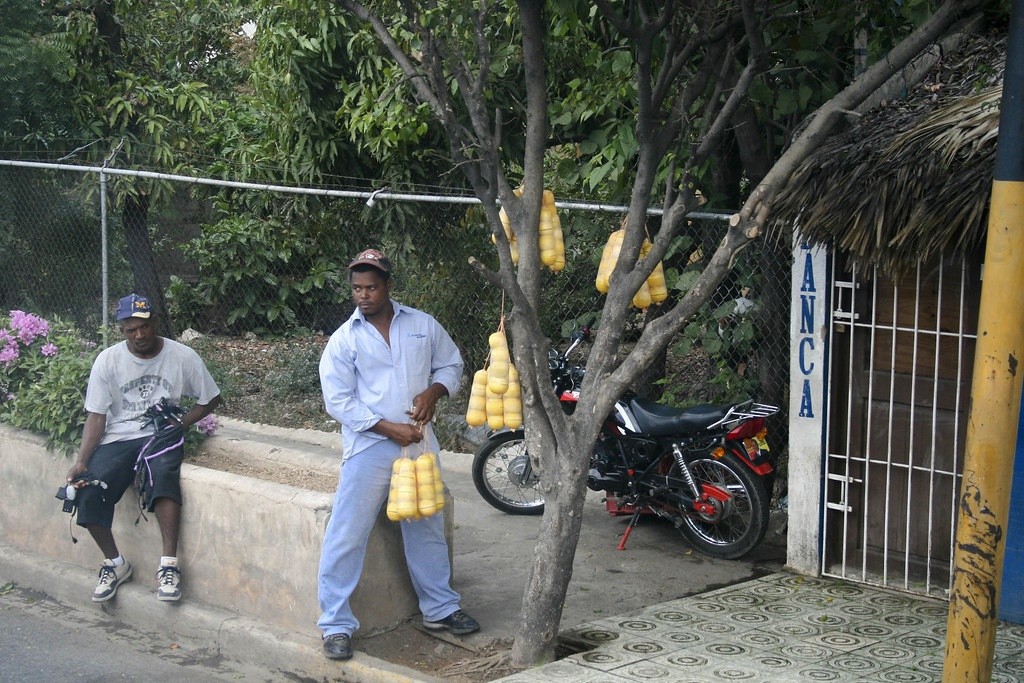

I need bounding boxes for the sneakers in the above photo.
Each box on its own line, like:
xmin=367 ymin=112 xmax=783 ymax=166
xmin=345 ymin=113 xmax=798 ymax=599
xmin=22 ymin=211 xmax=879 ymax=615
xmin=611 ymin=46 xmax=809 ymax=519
xmin=92 ymin=559 xmax=133 ymax=602
xmin=423 ymin=608 xmax=480 ymax=634
xmin=323 ymin=634 xmax=353 ymax=659
xmin=155 ymin=564 xmax=182 ymax=601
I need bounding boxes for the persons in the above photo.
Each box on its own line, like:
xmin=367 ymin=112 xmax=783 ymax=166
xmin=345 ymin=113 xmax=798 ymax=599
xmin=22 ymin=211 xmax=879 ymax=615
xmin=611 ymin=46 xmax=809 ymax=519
xmin=318 ymin=249 xmax=480 ymax=660
xmin=66 ymin=294 xmax=222 ymax=603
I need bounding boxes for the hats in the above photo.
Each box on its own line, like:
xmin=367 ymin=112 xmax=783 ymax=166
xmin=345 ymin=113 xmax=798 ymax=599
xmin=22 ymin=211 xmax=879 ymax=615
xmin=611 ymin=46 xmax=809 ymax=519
xmin=116 ymin=293 xmax=153 ymax=321
xmin=349 ymin=249 xmax=391 ymax=277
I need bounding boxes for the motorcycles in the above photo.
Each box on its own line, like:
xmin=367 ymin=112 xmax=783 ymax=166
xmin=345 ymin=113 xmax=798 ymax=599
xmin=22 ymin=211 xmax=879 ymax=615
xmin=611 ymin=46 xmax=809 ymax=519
xmin=472 ymin=317 xmax=782 ymax=561
